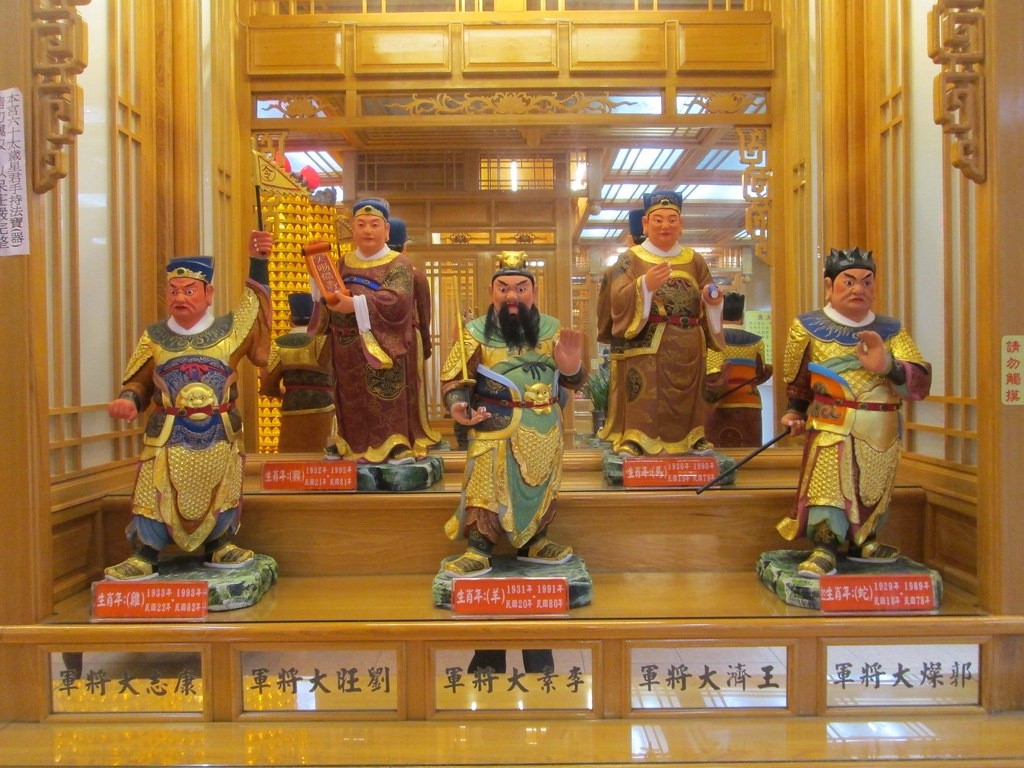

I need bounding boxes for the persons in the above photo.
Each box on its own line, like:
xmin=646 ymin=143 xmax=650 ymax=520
xmin=775 ymin=240 xmax=932 ymax=579
xmin=594 ymin=188 xmax=728 ymax=460
xmin=258 ymin=215 xmax=776 ymax=452
xmin=304 ymin=194 xmax=421 ymax=468
xmin=103 ymin=227 xmax=275 ymax=586
xmin=435 ymin=249 xmax=590 ymax=577
